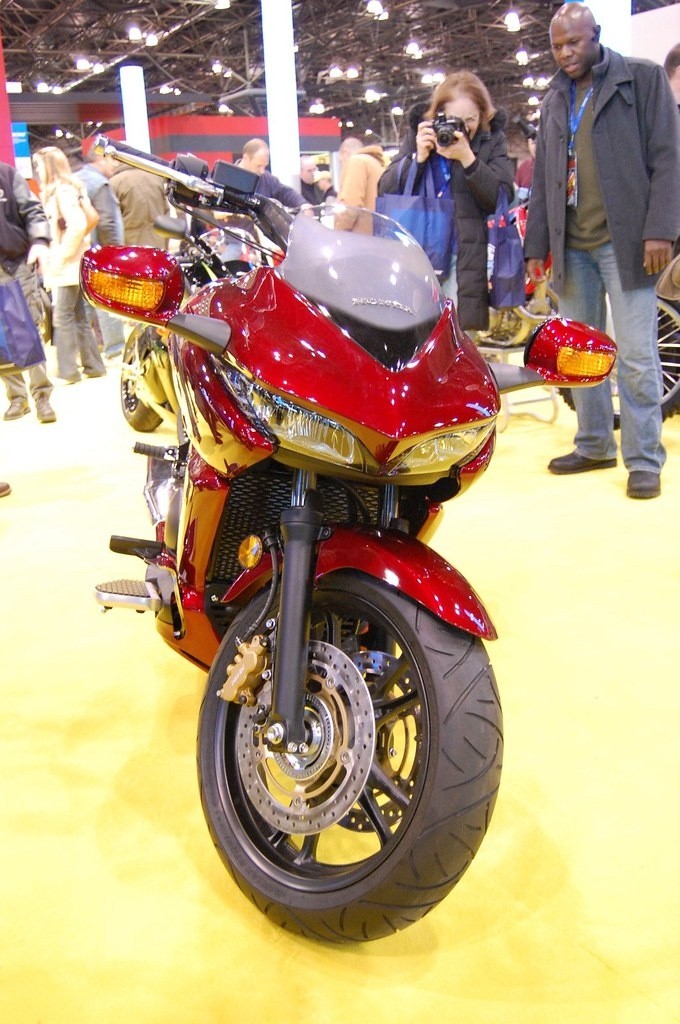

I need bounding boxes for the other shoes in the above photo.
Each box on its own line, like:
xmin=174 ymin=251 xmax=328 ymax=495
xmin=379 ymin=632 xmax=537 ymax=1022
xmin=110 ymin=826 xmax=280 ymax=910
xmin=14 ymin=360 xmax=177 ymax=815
xmin=50 ymin=376 xmax=81 ymax=387
xmin=0 ymin=483 xmax=12 ymax=497
xmin=36 ymin=395 xmax=56 ymax=422
xmin=3 ymin=400 xmax=32 ymax=419
xmin=81 ymin=369 xmax=107 ymax=380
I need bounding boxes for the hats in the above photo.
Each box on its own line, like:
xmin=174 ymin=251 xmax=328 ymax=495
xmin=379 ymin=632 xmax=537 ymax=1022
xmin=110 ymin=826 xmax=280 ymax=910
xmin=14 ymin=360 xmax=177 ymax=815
xmin=313 ymin=171 xmax=333 ymax=182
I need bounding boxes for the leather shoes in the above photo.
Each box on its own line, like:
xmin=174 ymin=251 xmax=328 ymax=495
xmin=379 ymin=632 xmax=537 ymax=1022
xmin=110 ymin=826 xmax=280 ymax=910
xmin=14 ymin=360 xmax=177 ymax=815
xmin=548 ymin=451 xmax=619 ymax=475
xmin=627 ymin=471 xmax=662 ymax=498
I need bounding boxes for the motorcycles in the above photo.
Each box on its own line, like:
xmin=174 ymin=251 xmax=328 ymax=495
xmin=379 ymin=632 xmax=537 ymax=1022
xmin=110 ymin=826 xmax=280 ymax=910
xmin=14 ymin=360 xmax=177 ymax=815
xmin=76 ymin=133 xmax=622 ymax=953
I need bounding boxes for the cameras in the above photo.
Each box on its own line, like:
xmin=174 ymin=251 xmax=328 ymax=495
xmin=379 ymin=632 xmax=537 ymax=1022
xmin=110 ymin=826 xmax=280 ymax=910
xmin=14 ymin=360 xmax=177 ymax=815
xmin=431 ymin=113 xmax=466 ymax=146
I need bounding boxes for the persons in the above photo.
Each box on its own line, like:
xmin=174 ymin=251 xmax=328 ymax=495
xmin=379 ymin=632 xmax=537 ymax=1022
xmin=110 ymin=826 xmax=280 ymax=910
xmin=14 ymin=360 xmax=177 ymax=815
xmin=522 ymin=2 xmax=680 ymax=499
xmin=0 ymin=47 xmax=680 ymax=494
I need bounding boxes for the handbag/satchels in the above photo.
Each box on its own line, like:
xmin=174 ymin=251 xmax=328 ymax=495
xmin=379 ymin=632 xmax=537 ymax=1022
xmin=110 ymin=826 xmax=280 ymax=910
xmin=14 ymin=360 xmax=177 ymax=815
xmin=368 ymin=152 xmax=458 ymax=285
xmin=485 ymin=186 xmax=529 ymax=310
